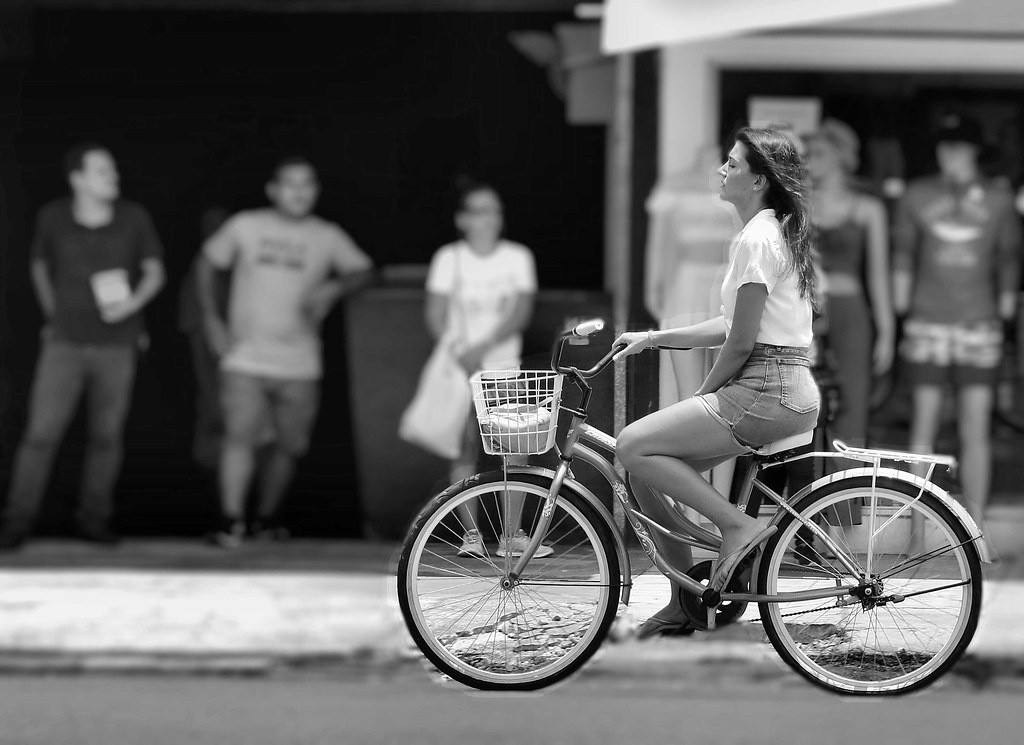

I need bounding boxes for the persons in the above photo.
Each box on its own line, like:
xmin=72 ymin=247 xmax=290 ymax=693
xmin=800 ymin=122 xmax=894 ymax=560
xmin=0 ymin=148 xmax=169 ymax=553
xmin=609 ymin=124 xmax=824 ymax=639
xmin=888 ymin=124 xmax=1020 ymax=559
xmin=418 ymin=180 xmax=555 ymax=560
xmin=193 ymin=155 xmax=377 ymax=552
xmin=641 ymin=146 xmax=751 ymax=548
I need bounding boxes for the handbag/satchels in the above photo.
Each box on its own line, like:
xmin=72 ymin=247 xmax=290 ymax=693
xmin=399 ymin=333 xmax=473 ymax=459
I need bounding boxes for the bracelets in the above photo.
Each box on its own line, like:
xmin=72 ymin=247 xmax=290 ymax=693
xmin=647 ymin=328 xmax=658 ymax=350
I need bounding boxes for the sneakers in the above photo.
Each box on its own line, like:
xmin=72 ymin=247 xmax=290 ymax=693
xmin=496 ymin=529 xmax=555 ymax=558
xmin=457 ymin=528 xmax=485 ymax=557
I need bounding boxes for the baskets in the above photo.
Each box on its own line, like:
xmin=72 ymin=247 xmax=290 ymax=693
xmin=470 ymin=371 xmax=566 ymax=455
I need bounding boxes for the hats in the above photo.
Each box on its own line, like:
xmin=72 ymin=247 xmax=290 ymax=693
xmin=929 ymin=110 xmax=981 ymax=143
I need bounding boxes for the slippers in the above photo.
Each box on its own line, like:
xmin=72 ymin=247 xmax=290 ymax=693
xmin=709 ymin=524 xmax=778 ymax=593
xmin=640 ymin=618 xmax=704 ymax=639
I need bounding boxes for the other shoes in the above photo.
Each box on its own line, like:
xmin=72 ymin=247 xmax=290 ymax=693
xmin=219 ymin=514 xmax=242 ymax=546
xmin=794 ymin=543 xmax=822 ymax=567
xmin=77 ymin=524 xmax=115 ymax=541
xmin=0 ymin=519 xmax=26 ymax=549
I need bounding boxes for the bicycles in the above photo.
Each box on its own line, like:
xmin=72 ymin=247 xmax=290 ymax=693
xmin=397 ymin=318 xmax=993 ymax=697
